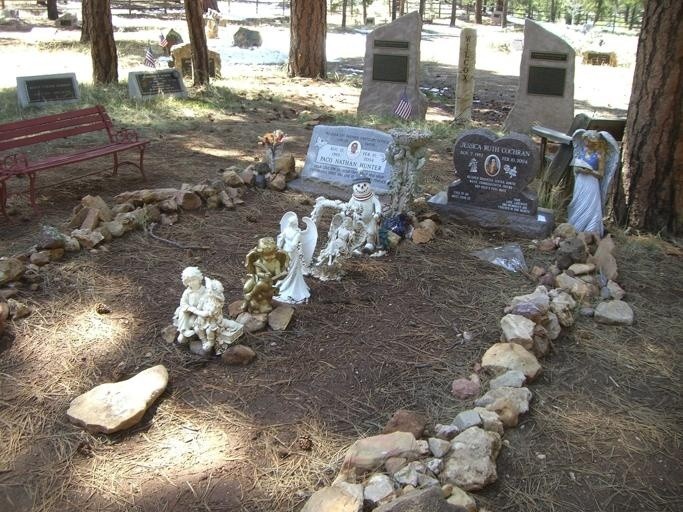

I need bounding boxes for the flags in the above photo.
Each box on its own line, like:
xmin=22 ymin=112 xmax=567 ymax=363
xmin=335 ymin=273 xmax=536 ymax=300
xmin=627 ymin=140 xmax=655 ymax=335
xmin=159 ymin=34 xmax=168 ymax=47
xmin=394 ymin=91 xmax=412 ymax=119
xmin=146 ymin=47 xmax=155 ymax=67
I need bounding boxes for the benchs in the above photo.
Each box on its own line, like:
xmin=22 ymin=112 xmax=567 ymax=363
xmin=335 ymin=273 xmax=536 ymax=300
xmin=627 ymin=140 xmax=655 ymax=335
xmin=0 ymin=100 xmax=154 ymax=225
xmin=531 ymin=114 xmax=626 ymax=208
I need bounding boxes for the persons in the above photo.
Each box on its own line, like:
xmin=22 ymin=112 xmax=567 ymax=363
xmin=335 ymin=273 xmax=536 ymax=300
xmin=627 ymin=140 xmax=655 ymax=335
xmin=272 ymin=217 xmax=311 ymax=305
xmin=487 ymin=158 xmax=499 ymax=175
xmin=192 ymin=277 xmax=224 ymax=352
xmin=567 ymin=129 xmax=609 ymax=238
xmin=314 ymin=216 xmax=353 ymax=266
xmin=241 ymin=237 xmax=288 ymax=310
xmin=177 ymin=267 xmax=207 ymax=343
xmin=351 ymin=143 xmax=358 ymax=153
